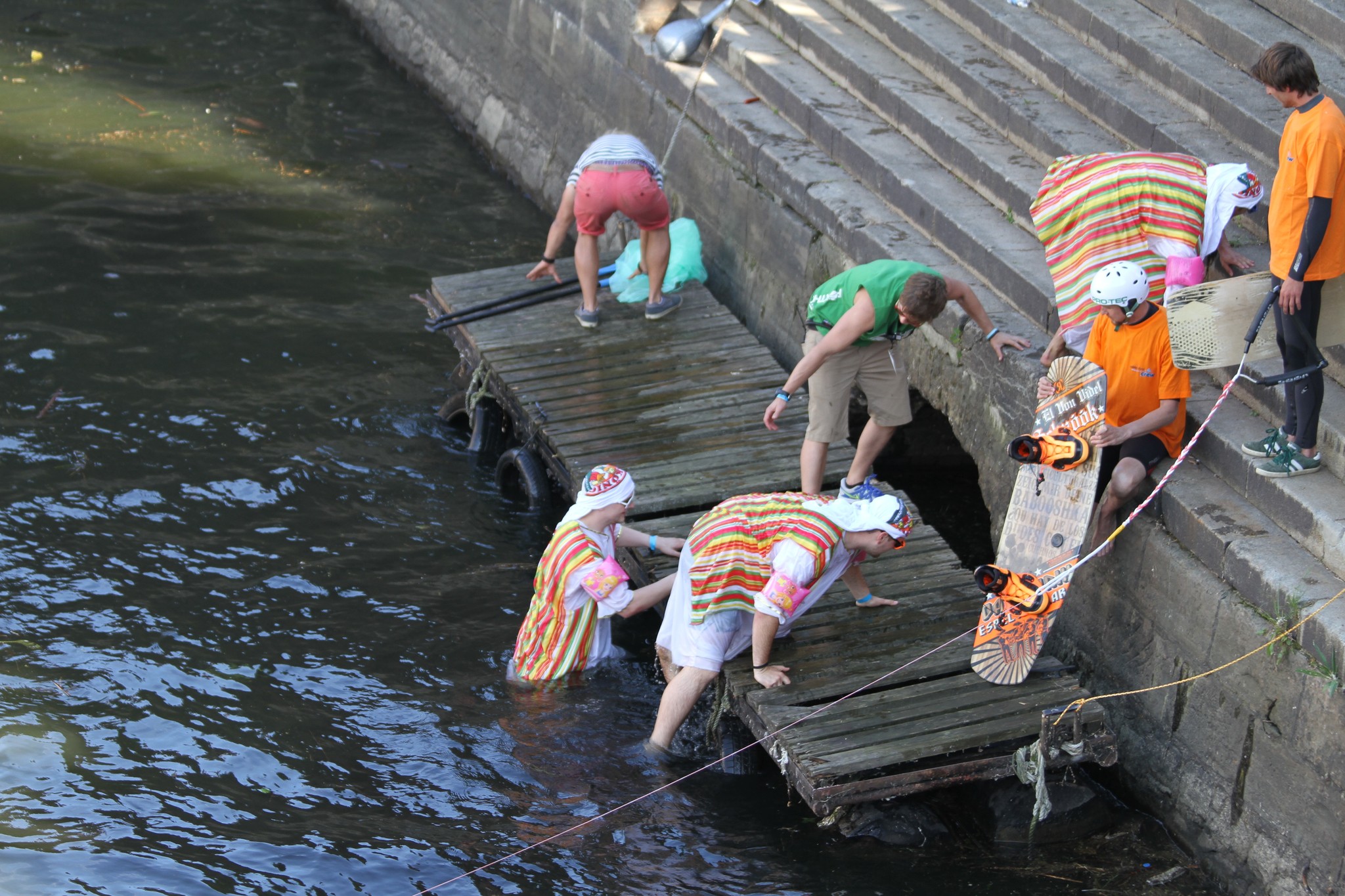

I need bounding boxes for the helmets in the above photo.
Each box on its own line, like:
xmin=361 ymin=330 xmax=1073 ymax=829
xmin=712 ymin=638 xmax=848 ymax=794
xmin=1090 ymin=260 xmax=1150 ymax=307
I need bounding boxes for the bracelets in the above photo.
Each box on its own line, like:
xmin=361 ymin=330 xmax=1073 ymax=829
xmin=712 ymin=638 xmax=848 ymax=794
xmin=636 ymin=262 xmax=649 ymax=275
xmin=649 ymin=535 xmax=657 ymax=550
xmin=855 ymin=593 xmax=872 ymax=604
xmin=752 ymin=661 xmax=769 ymax=669
xmin=776 ymin=394 xmax=789 ymax=403
xmin=542 ymin=254 xmax=555 ymax=264
xmin=986 ymin=328 xmax=1000 ymax=341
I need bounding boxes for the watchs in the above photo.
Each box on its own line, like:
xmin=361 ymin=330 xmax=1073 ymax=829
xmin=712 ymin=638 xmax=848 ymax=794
xmin=774 ymin=387 xmax=792 ymax=401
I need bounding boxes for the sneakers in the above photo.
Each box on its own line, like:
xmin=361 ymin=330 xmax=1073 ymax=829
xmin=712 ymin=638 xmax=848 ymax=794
xmin=1256 ymin=440 xmax=1322 ymax=480
xmin=1241 ymin=425 xmax=1291 ymax=458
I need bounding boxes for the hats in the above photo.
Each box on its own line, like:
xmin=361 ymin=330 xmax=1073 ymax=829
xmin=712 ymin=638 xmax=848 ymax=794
xmin=1200 ymin=162 xmax=1264 ymax=263
xmin=802 ymin=494 xmax=914 ymax=539
xmin=555 ymin=464 xmax=635 ymax=529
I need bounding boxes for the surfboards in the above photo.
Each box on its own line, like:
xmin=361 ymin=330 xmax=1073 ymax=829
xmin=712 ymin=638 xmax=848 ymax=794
xmin=969 ymin=356 xmax=1111 ymax=687
xmin=1165 ymin=261 xmax=1345 ymax=367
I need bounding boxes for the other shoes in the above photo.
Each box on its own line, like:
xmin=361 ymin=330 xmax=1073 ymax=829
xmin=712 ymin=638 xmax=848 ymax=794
xmin=974 ymin=564 xmax=1050 ymax=627
xmin=1006 ymin=427 xmax=1089 ymax=472
xmin=838 ymin=474 xmax=885 ymax=505
xmin=644 ymin=294 xmax=683 ymax=319
xmin=574 ymin=302 xmax=599 ymax=328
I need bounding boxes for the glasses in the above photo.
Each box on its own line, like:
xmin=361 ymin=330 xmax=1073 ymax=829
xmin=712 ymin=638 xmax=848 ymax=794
xmin=892 ymin=537 xmax=905 ymax=549
xmin=894 ymin=300 xmax=924 ymax=327
xmin=617 ymin=490 xmax=634 ymax=509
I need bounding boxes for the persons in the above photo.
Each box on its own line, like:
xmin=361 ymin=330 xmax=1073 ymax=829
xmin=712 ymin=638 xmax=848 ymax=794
xmin=1242 ymin=40 xmax=1345 ymax=478
xmin=763 ymin=258 xmax=1030 ymax=499
xmin=1036 ymin=259 xmax=1191 ymax=559
xmin=645 ymin=469 xmax=915 ymax=760
xmin=1028 ymin=151 xmax=1265 ymax=367
xmin=524 ymin=126 xmax=685 ymax=332
xmin=506 ymin=463 xmax=687 ymax=686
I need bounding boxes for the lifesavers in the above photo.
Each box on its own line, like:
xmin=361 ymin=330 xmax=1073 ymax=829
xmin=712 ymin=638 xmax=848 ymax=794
xmin=488 ymin=437 xmax=548 ymax=527
xmin=697 ymin=731 xmax=757 ymax=779
xmin=432 ymin=396 xmax=498 ymax=457
